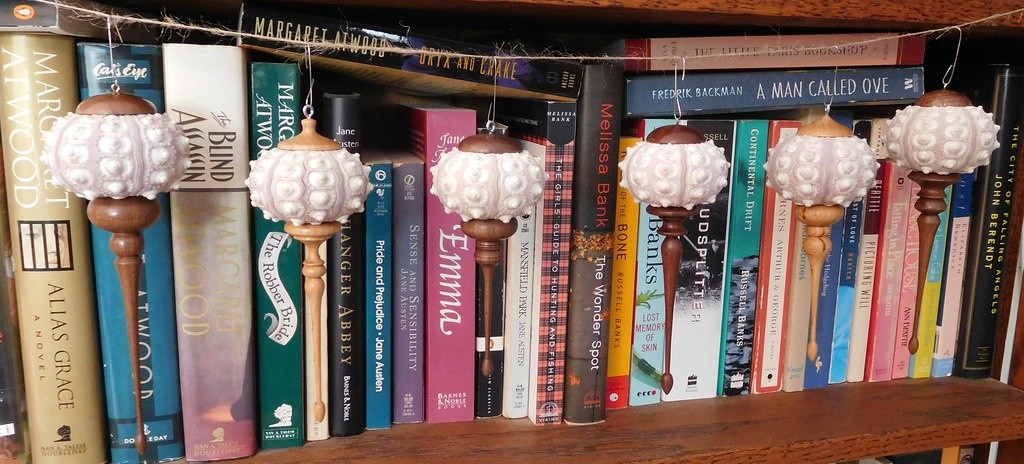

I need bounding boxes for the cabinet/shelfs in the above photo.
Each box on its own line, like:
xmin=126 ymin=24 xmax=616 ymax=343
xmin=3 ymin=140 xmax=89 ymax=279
xmin=0 ymin=0 xmax=1024 ymax=464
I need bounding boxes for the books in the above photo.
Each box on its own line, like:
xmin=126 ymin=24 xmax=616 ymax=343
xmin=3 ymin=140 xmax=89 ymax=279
xmin=0 ymin=0 xmax=1024 ymax=464
